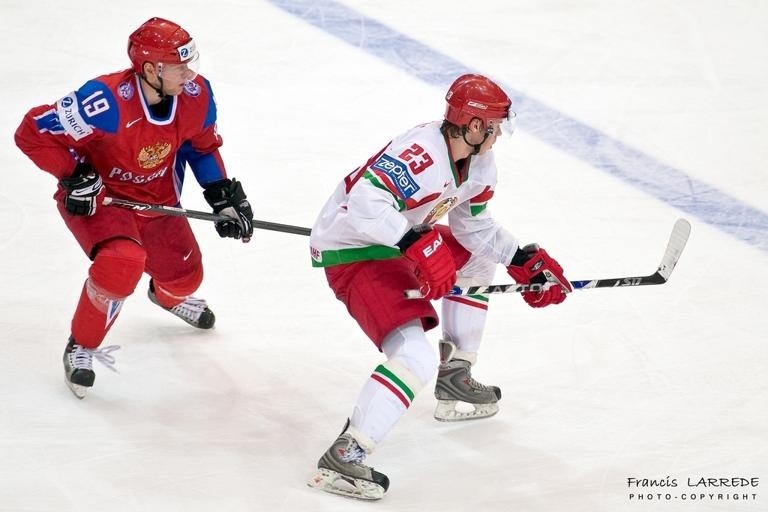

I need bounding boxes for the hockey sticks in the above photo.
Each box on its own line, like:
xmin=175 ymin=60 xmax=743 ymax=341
xmin=404 ymin=219 xmax=692 ymax=301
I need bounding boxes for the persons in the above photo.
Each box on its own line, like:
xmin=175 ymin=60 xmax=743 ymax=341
xmin=310 ymin=74 xmax=572 ymax=492
xmin=15 ymin=17 xmax=252 ymax=387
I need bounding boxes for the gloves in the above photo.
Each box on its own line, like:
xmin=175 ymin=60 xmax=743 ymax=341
xmin=61 ymin=162 xmax=107 ymax=216
xmin=393 ymin=228 xmax=457 ymax=301
xmin=506 ymin=242 xmax=574 ymax=309
xmin=202 ymin=177 xmax=254 ymax=244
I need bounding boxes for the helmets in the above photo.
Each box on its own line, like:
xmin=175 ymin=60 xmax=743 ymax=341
xmin=127 ymin=17 xmax=195 ymax=75
xmin=443 ymin=73 xmax=513 ymax=129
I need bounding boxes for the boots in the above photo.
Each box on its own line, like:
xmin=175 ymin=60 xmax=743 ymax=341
xmin=317 ymin=416 xmax=390 ymax=492
xmin=435 ymin=340 xmax=502 ymax=405
xmin=63 ymin=335 xmax=96 ymax=387
xmin=148 ymin=277 xmax=216 ymax=329
